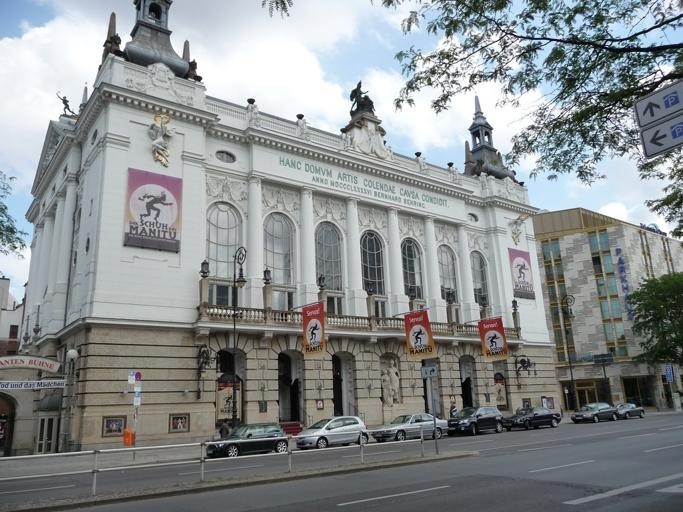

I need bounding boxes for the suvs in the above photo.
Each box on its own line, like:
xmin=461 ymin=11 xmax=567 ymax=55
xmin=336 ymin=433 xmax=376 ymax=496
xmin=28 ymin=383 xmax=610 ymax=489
xmin=447 ymin=406 xmax=503 ymax=437
xmin=206 ymin=422 xmax=289 ymax=458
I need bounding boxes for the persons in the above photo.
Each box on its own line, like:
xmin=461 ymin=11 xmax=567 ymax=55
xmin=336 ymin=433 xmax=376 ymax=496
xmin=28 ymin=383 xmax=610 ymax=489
xmin=109 ymin=420 xmax=121 ymax=432
xmin=56 ymin=92 xmax=75 ymax=114
xmin=151 ymin=115 xmax=168 ymax=166
xmin=381 ymin=359 xmax=400 ymax=407
xmin=174 ymin=418 xmax=186 ymax=428
xmin=354 ymin=80 xmax=369 ymax=105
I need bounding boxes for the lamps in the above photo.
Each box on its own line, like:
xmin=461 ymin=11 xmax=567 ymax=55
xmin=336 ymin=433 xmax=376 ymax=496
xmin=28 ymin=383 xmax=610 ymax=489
xmin=236 ymin=264 xmax=247 ymax=289
xmin=568 ymin=305 xmax=576 ymax=322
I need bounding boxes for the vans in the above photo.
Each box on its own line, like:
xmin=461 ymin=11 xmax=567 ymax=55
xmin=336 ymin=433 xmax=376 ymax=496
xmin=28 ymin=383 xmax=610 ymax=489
xmin=294 ymin=415 xmax=370 ymax=450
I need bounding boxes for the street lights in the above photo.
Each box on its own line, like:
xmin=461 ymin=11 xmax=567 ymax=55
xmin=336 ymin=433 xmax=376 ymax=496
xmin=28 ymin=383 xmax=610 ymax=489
xmin=560 ymin=294 xmax=576 ymax=414
xmin=60 ymin=349 xmax=78 ymax=453
xmin=229 ymin=246 xmax=247 ymax=429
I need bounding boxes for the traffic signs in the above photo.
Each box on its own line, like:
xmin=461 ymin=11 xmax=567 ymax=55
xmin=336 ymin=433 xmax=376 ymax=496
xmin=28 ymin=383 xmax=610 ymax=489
xmin=634 ymin=81 xmax=683 ymax=128
xmin=641 ymin=113 xmax=683 ymax=158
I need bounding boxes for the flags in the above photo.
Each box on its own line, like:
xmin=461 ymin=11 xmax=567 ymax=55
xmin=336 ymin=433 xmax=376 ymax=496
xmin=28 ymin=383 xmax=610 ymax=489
xmin=405 ymin=311 xmax=437 ymax=361
xmin=477 ymin=317 xmax=508 ymax=361
xmin=302 ymin=304 xmax=324 ymax=354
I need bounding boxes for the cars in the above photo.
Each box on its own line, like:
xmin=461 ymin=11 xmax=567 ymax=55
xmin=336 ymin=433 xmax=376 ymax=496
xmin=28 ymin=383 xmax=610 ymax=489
xmin=570 ymin=402 xmax=618 ymax=425
xmin=616 ymin=403 xmax=644 ymax=419
xmin=501 ymin=404 xmax=562 ymax=431
xmin=370 ymin=413 xmax=449 ymax=443
